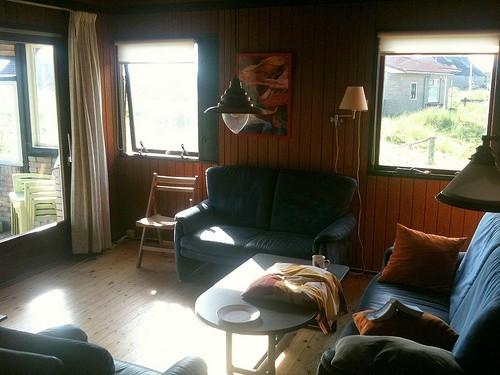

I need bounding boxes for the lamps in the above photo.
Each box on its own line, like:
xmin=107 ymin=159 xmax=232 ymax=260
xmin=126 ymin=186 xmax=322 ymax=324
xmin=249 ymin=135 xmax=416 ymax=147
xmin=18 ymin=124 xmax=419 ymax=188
xmin=204 ymin=8 xmax=267 ymax=134
xmin=436 ymin=135 xmax=500 ymax=213
xmin=331 ymin=86 xmax=368 ymax=125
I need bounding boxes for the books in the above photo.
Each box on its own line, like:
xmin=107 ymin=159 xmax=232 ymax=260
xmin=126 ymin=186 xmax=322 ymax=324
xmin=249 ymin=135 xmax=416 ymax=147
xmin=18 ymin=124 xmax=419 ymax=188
xmin=364 ymin=298 xmax=424 ymax=322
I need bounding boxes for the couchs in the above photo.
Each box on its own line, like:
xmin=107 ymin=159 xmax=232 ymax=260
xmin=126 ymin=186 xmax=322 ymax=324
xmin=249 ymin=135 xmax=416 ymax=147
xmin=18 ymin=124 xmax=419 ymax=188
xmin=316 ymin=212 xmax=500 ymax=375
xmin=0 ymin=323 xmax=207 ymax=375
xmin=173 ymin=165 xmax=359 ymax=290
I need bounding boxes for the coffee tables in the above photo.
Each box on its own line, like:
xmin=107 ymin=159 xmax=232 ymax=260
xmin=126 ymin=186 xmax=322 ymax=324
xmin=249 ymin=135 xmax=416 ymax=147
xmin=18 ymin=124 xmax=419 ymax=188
xmin=195 ymin=254 xmax=350 ymax=375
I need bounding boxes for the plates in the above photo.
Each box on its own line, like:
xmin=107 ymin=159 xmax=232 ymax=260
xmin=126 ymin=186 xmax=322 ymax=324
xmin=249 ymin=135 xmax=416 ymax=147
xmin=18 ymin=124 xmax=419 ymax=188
xmin=217 ymin=304 xmax=261 ymax=324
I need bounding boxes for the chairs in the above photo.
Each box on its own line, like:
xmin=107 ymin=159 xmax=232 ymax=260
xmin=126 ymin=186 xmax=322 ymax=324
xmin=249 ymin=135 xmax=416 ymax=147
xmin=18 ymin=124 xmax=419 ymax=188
xmin=135 ymin=172 xmax=198 ymax=267
xmin=9 ymin=173 xmax=57 ymax=235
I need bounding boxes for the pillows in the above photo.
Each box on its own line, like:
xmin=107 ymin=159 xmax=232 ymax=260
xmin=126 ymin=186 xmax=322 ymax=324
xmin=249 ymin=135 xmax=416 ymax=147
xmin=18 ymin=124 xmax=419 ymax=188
xmin=351 ymin=309 xmax=459 ymax=346
xmin=378 ymin=223 xmax=468 ymax=287
xmin=241 ymin=263 xmax=348 ymax=334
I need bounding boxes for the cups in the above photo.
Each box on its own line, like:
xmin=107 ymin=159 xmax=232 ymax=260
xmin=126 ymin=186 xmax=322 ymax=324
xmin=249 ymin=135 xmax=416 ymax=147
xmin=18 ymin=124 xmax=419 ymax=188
xmin=312 ymin=255 xmax=330 ymax=269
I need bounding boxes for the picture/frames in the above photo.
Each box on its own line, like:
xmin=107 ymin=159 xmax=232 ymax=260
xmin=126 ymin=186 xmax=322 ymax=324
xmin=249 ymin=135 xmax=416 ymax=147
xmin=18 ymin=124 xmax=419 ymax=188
xmin=236 ymin=53 xmax=292 ymax=139
